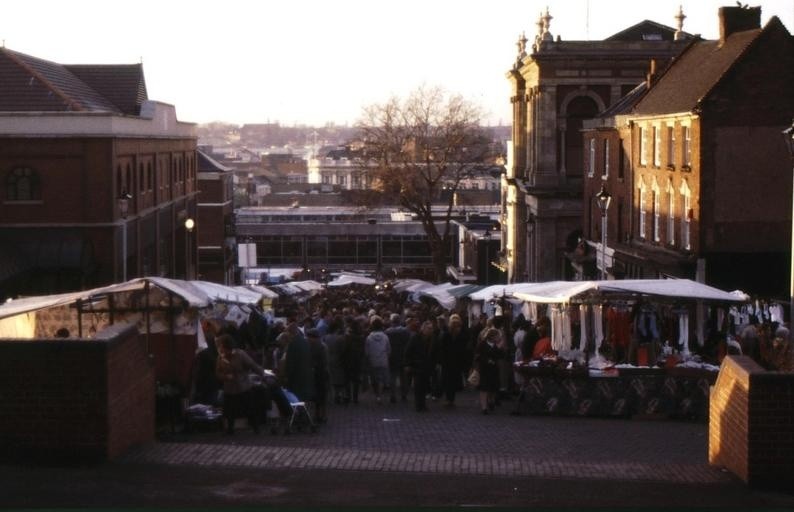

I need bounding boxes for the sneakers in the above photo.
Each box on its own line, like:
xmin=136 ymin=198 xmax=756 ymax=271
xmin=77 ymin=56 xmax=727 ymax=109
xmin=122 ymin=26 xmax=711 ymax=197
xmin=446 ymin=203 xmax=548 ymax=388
xmin=334 ymin=388 xmax=454 ymax=413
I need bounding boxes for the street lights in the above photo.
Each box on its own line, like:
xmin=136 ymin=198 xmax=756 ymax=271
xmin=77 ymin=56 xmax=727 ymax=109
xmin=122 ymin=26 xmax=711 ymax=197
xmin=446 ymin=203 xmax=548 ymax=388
xmin=117 ymin=186 xmax=131 ymax=283
xmin=596 ymin=183 xmax=611 ymax=284
xmin=185 ymin=217 xmax=196 ymax=280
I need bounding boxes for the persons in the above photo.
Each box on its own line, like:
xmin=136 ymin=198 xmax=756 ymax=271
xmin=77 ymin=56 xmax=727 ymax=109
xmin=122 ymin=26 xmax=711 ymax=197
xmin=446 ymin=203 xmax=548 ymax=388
xmin=51 ymin=262 xmax=794 ymax=435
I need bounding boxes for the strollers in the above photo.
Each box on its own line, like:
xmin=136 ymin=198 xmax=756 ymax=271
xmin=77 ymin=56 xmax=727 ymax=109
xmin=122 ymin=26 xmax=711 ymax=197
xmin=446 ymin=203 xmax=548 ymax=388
xmin=251 ymin=373 xmax=317 ymax=435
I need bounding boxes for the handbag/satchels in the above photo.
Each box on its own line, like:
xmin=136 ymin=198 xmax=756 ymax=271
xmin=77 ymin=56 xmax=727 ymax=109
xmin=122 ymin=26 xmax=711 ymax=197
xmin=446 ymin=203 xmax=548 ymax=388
xmin=467 ymin=370 xmax=481 ymax=386
